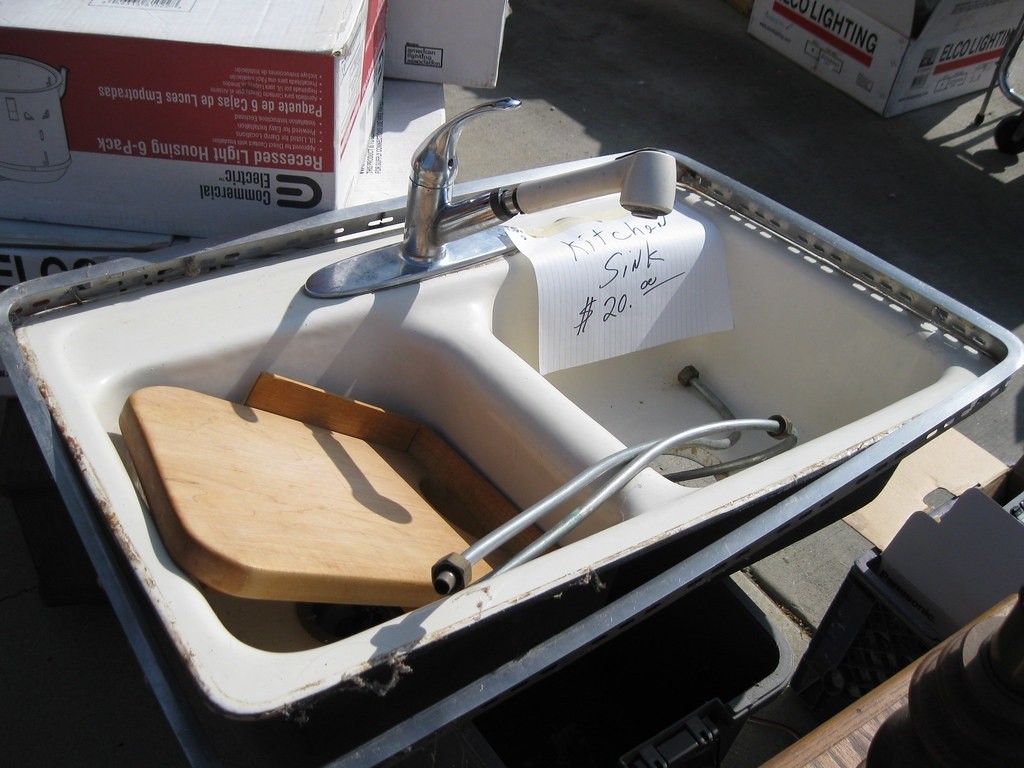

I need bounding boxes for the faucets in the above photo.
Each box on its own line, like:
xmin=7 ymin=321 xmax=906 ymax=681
xmin=401 ymin=96 xmax=679 ymax=269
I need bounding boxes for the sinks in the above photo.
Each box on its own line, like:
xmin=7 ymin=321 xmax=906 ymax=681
xmin=81 ymin=302 xmax=655 ymax=661
xmin=448 ymin=214 xmax=978 ymax=493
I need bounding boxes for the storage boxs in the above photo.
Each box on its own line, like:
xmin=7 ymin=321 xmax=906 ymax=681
xmin=377 ymin=0 xmax=510 ymax=89
xmin=0 ymin=0 xmax=391 ymax=241
xmin=746 ymin=0 xmax=1024 ymax=116
xmin=790 ymin=455 xmax=1024 ymax=722
